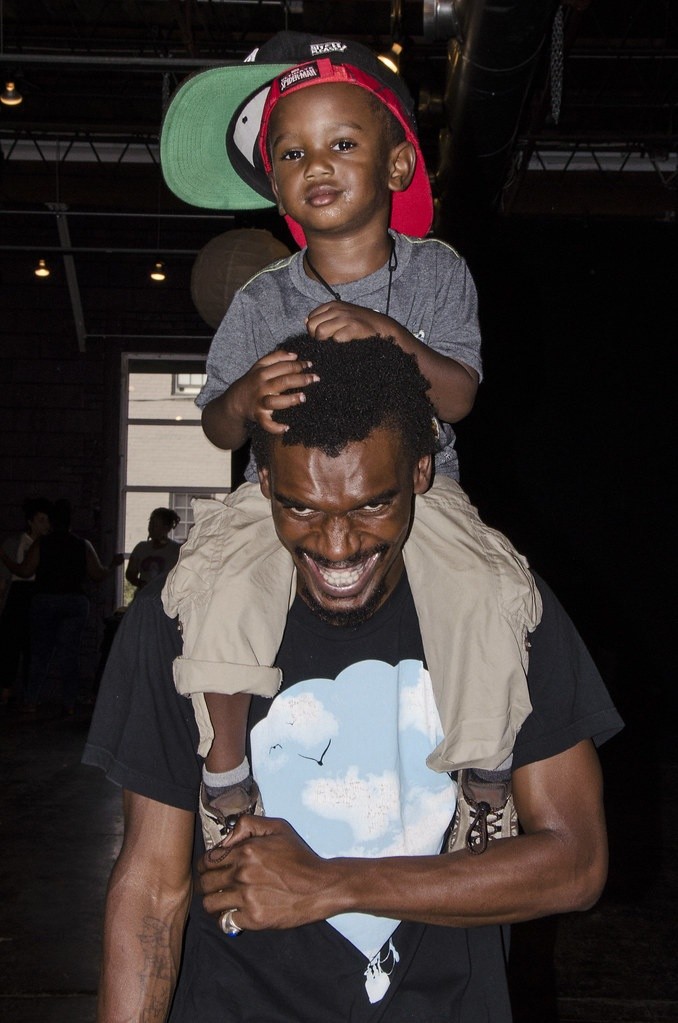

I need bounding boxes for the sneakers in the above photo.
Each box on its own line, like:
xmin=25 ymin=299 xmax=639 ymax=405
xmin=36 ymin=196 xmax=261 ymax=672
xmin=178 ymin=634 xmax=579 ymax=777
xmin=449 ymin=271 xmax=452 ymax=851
xmin=444 ymin=778 xmax=523 ymax=855
xmin=195 ymin=778 xmax=263 ymax=855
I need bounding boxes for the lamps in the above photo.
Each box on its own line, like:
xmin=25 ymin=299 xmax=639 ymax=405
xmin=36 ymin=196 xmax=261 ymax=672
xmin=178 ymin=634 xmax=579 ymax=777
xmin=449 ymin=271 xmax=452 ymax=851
xmin=149 ymin=259 xmax=167 ymax=281
xmin=35 ymin=259 xmax=50 ymax=277
xmin=1 ymin=82 xmax=23 ymax=106
xmin=378 ymin=41 xmax=402 ymax=72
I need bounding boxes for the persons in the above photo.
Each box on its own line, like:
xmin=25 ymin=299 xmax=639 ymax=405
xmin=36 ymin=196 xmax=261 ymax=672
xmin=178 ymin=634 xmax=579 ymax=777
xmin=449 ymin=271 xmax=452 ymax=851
xmin=161 ymin=30 xmax=544 ymax=854
xmin=125 ymin=508 xmax=181 ymax=607
xmin=97 ymin=334 xmax=627 ymax=1023
xmin=0 ymin=497 xmax=124 ymax=716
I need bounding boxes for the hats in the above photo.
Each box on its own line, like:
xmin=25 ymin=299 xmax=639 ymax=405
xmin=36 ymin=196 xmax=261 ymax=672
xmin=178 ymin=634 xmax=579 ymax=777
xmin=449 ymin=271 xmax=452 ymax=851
xmin=161 ymin=32 xmax=419 ymax=211
xmin=256 ymin=58 xmax=435 ymax=247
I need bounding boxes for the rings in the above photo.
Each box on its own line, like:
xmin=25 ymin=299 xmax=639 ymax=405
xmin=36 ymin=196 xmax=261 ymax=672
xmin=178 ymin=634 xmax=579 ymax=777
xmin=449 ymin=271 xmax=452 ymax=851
xmin=221 ymin=908 xmax=243 ymax=938
